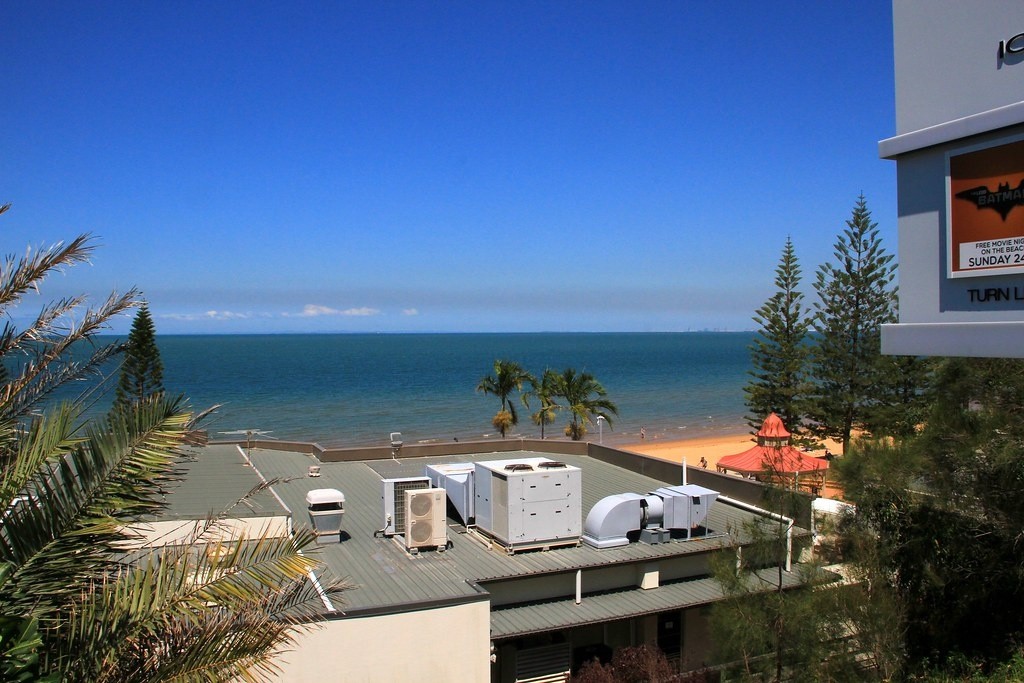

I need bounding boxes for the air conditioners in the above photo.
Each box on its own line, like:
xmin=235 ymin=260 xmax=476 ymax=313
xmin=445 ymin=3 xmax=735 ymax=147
xmin=404 ymin=488 xmax=447 ymax=548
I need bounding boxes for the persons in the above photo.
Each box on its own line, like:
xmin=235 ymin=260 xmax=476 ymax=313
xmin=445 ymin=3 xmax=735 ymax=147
xmin=825 ymin=450 xmax=828 ymax=454
xmin=454 ymin=437 xmax=458 ymax=442
xmin=697 ymin=457 xmax=707 ymax=468
xmin=721 ymin=468 xmax=727 ymax=473
xmin=641 ymin=427 xmax=645 ymax=438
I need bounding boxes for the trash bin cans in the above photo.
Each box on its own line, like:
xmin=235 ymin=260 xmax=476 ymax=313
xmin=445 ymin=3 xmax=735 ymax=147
xmin=306 ymin=488 xmax=346 ymax=545
